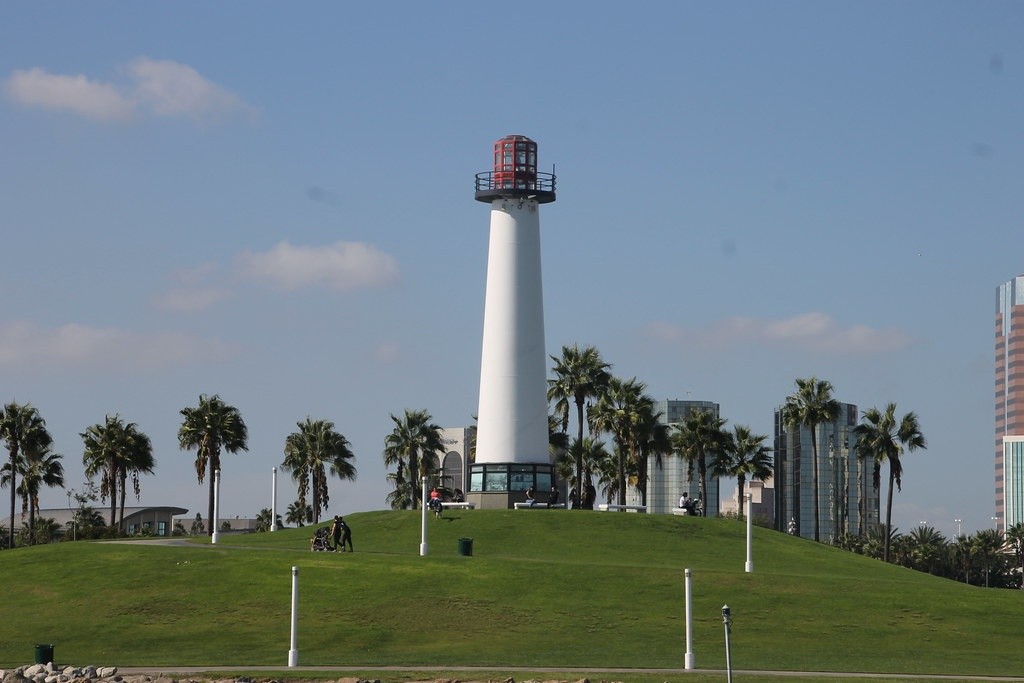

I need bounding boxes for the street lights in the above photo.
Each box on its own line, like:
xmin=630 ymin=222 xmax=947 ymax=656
xmin=955 ymin=519 xmax=963 ymax=539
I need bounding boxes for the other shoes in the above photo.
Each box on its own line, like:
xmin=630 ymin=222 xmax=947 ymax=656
xmin=332 ymin=549 xmax=337 ymax=552
xmin=349 ymin=549 xmax=354 ymax=552
xmin=342 ymin=548 xmax=346 ymax=551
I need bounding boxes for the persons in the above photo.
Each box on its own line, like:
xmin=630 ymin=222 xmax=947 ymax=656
xmin=585 ymin=482 xmax=596 ymax=511
xmin=548 ymin=486 xmax=559 ymax=508
xmin=330 ymin=515 xmax=345 ymax=552
xmin=525 ymin=486 xmax=536 ymax=509
xmin=339 ymin=517 xmax=353 ymax=552
xmin=429 ymin=487 xmax=463 ymax=520
xmin=680 ymin=492 xmax=698 ymax=516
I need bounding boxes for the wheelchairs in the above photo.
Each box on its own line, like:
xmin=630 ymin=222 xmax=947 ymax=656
xmin=309 ymin=531 xmax=335 ymax=551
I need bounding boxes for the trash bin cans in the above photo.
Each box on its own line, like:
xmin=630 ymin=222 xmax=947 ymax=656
xmin=458 ymin=537 xmax=474 ymax=556
xmin=35 ymin=643 xmax=54 ymax=664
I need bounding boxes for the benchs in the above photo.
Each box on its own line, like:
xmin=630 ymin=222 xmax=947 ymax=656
xmin=514 ymin=502 xmax=565 ymax=509
xmin=672 ymin=508 xmax=703 ymax=516
xmin=599 ymin=504 xmax=646 ymax=512
xmin=427 ymin=502 xmax=476 ymax=509
